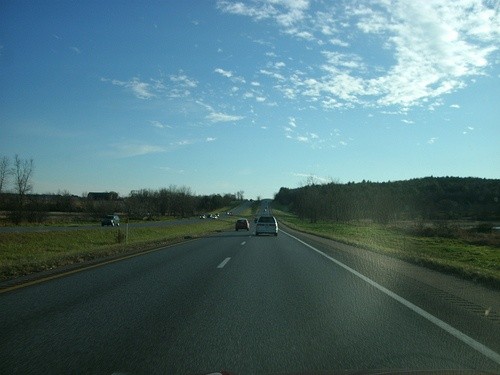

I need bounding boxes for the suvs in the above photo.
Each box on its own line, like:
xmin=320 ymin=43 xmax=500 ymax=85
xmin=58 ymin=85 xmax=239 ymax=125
xmin=101 ymin=214 xmax=120 ymax=226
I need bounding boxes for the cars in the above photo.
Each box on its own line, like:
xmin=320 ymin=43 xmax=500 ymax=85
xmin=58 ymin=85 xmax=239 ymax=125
xmin=255 ymin=216 xmax=279 ymax=236
xmin=235 ymin=218 xmax=249 ymax=231
xmin=199 ymin=211 xmax=232 ymax=220
